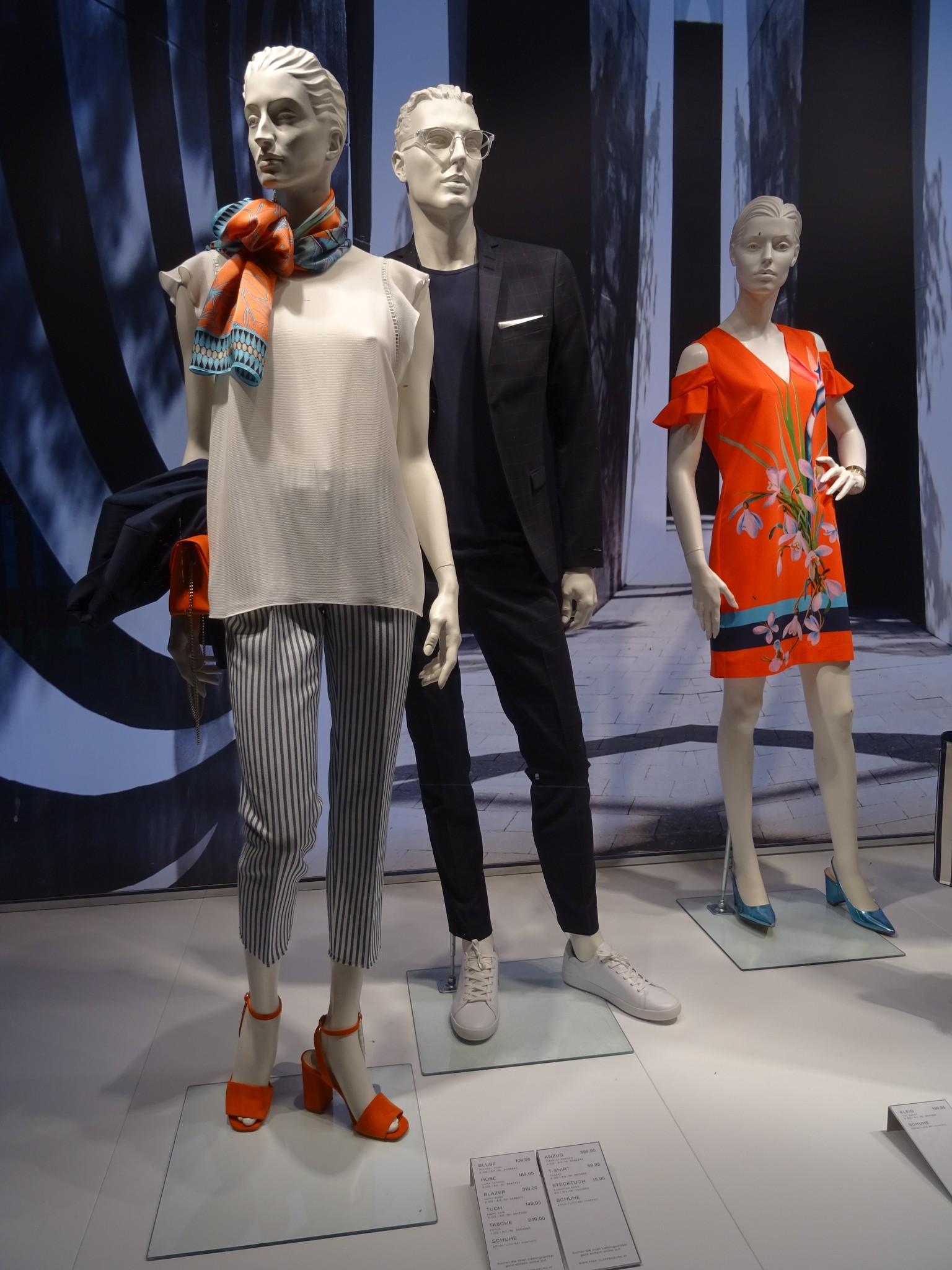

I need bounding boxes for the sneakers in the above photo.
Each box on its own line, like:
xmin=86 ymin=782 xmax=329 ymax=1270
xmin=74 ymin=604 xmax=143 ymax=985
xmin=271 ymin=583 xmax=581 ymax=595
xmin=564 ymin=940 xmax=682 ymax=1022
xmin=449 ymin=938 xmax=498 ymax=1042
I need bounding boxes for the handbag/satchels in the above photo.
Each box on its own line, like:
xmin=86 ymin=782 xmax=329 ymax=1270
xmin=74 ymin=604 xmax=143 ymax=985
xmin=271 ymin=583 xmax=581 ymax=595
xmin=167 ymin=538 xmax=224 ymax=656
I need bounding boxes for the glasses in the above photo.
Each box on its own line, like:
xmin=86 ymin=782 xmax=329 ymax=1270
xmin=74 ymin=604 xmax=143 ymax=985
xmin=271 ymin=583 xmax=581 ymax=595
xmin=400 ymin=126 xmax=497 ymax=161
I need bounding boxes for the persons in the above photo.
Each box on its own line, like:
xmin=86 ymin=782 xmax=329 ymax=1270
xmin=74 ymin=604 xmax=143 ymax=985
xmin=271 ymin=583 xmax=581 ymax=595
xmin=378 ymin=84 xmax=683 ymax=1042
xmin=66 ymin=45 xmax=464 ymax=1142
xmin=653 ymin=197 xmax=896 ymax=937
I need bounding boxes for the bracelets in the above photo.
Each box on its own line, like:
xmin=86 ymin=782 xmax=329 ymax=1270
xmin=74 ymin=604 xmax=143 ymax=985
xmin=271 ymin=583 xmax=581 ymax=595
xmin=845 ymin=465 xmax=867 ymax=495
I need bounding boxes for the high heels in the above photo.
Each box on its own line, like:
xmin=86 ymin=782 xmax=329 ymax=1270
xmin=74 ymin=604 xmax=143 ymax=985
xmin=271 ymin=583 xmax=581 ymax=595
xmin=823 ymin=859 xmax=897 ymax=935
xmin=731 ymin=872 xmax=779 ymax=925
xmin=225 ymin=994 xmax=281 ymax=1131
xmin=299 ymin=1014 xmax=412 ymax=1141
